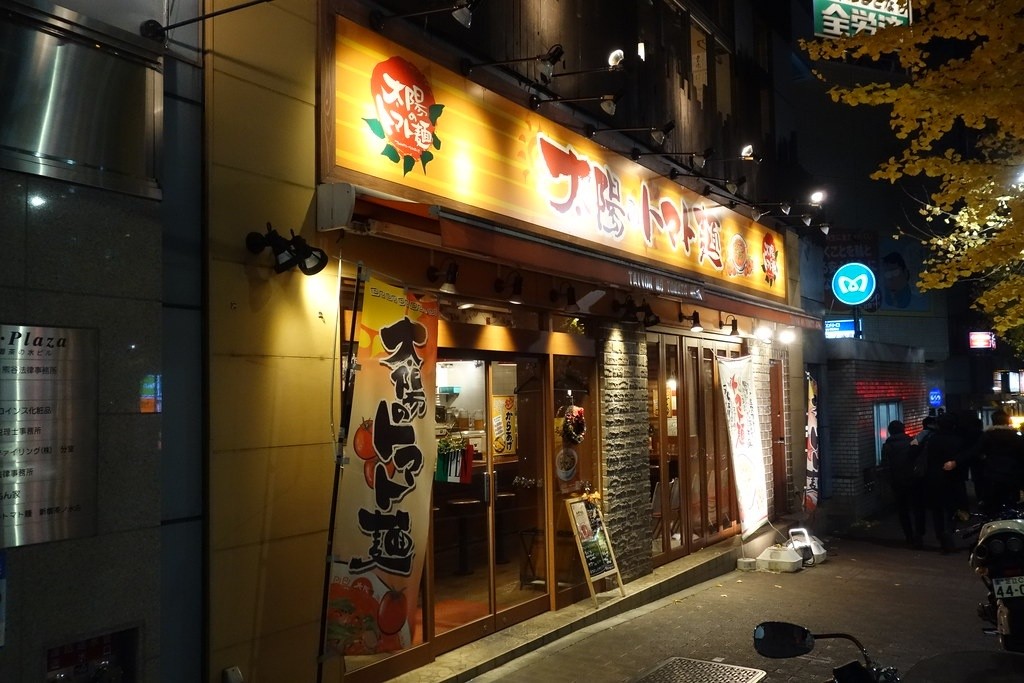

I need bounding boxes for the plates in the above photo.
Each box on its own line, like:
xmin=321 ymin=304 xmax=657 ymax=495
xmin=556 ymin=468 xmax=576 ymax=481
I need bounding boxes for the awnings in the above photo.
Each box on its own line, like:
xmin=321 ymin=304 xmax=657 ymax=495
xmin=364 ymin=195 xmax=822 ymax=331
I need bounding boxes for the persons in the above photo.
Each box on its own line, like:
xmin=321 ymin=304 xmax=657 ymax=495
xmin=909 ymin=410 xmax=1024 ymax=556
xmin=881 ymin=418 xmax=928 ymax=549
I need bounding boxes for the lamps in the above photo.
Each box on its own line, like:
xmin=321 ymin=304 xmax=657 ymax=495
xmin=381 ymin=3 xmax=473 ymax=29
xmin=540 ymin=49 xmax=628 ymax=85
xmin=775 ymin=222 xmax=830 ymax=235
xmin=703 ymin=183 xmax=771 ymax=222
xmin=529 ymin=94 xmax=618 ymax=115
xmin=549 ymin=282 xmax=580 ymax=313
xmin=631 ymin=146 xmax=722 ymax=169
xmin=460 ymin=44 xmax=565 ymax=78
xmin=245 ymin=220 xmax=328 ymax=275
xmin=729 ymin=197 xmax=791 ymax=215
xmin=669 ymin=167 xmax=746 ymax=195
xmin=766 ymin=214 xmax=812 ymax=227
xmin=719 ymin=315 xmax=740 ymax=336
xmin=637 ymin=297 xmax=661 ymax=328
xmin=426 ymin=256 xmax=458 ymax=294
xmin=707 ymin=144 xmax=771 ymax=167
xmin=678 ymin=310 xmax=703 ymax=333
xmin=495 ymin=270 xmax=524 ymax=304
xmin=585 ymin=120 xmax=676 ymax=145
xmin=753 ymin=190 xmax=828 ymax=210
xmin=610 ymin=295 xmax=639 ymax=324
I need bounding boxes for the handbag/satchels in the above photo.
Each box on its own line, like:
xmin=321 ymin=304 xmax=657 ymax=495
xmin=905 ymin=436 xmax=930 ymax=480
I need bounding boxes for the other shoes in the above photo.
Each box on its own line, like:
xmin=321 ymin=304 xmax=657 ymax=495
xmin=905 ymin=536 xmax=924 ymax=550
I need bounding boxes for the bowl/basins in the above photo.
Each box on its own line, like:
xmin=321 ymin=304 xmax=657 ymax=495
xmin=556 ymin=448 xmax=578 ymax=477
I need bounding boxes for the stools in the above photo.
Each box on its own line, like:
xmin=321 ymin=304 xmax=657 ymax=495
xmin=446 ymin=498 xmax=480 ymax=575
xmin=496 ymin=493 xmax=515 ymax=564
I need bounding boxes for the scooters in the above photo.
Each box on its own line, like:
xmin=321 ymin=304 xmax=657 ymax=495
xmin=954 ymin=490 xmax=1024 ymax=653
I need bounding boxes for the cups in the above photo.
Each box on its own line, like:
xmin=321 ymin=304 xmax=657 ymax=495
xmin=474 ymin=410 xmax=483 ymax=430
xmin=459 ymin=411 xmax=469 ymax=430
